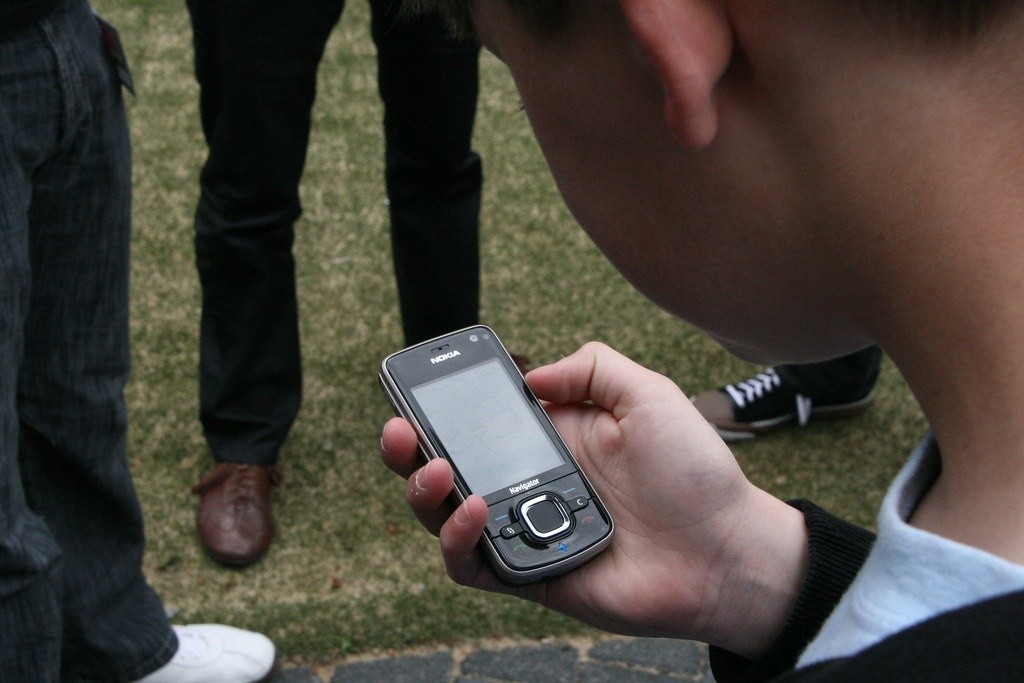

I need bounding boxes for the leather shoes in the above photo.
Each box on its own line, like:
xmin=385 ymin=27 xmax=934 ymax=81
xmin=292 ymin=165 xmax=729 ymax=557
xmin=192 ymin=461 xmax=282 ymax=567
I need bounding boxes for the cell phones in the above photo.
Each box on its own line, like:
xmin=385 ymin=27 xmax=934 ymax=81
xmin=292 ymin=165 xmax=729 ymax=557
xmin=378 ymin=325 xmax=615 ymax=585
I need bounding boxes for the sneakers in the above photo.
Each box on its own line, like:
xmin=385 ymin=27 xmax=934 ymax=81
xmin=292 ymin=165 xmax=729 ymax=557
xmin=689 ymin=345 xmax=883 ymax=441
xmin=132 ymin=624 xmax=275 ymax=683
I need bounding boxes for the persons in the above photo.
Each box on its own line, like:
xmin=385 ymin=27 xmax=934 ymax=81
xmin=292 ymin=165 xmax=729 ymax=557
xmin=184 ymin=1 xmax=484 ymax=569
xmin=464 ymin=0 xmax=1024 ymax=683
xmin=685 ymin=354 xmax=879 ymax=438
xmin=0 ymin=0 xmax=277 ymax=683
